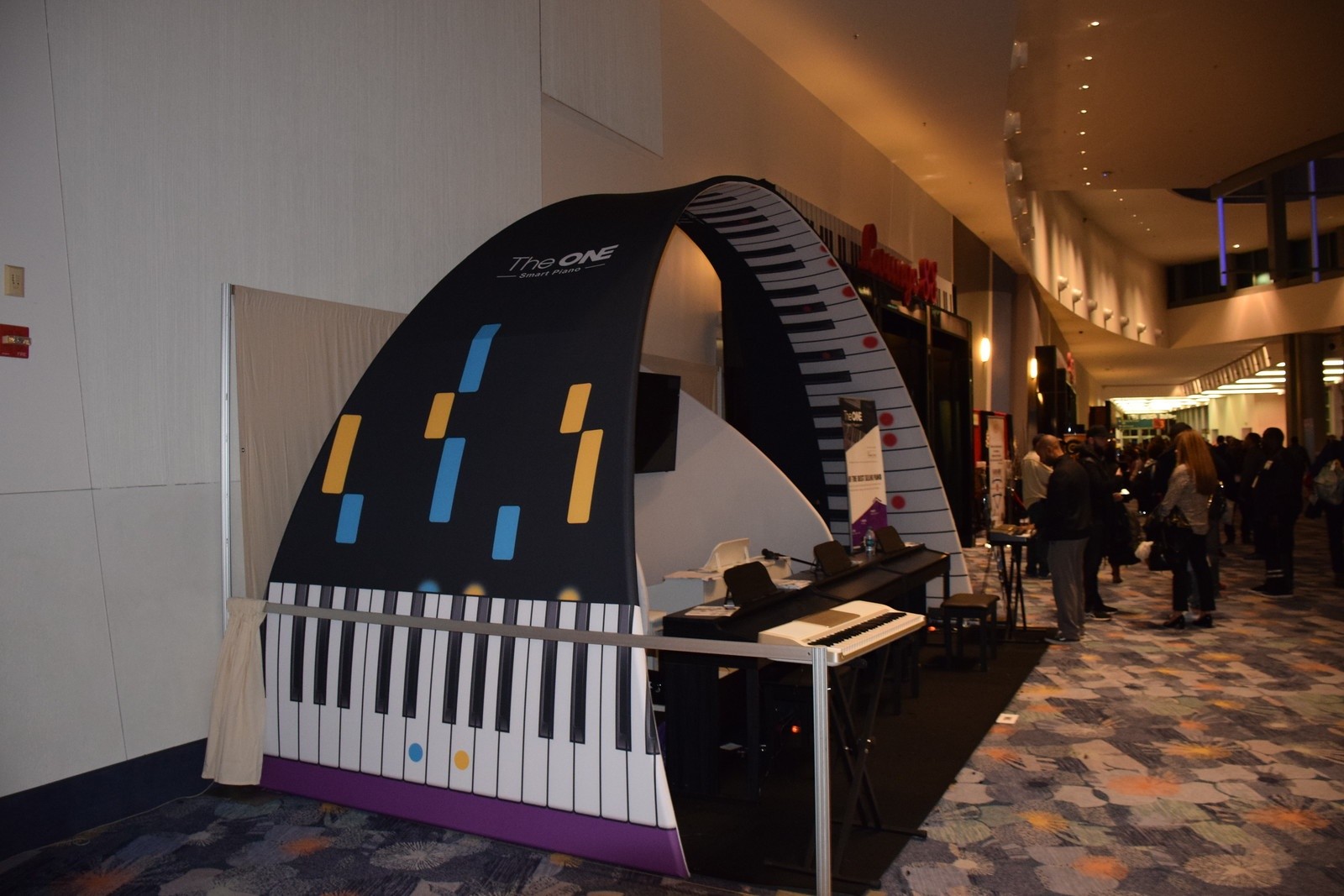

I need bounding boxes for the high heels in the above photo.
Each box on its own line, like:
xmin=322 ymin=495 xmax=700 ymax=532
xmin=1163 ymin=614 xmax=1186 ymax=629
xmin=1192 ymin=613 xmax=1213 ymax=628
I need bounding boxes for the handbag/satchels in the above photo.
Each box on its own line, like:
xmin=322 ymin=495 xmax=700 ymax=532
xmin=1314 ymin=459 xmax=1344 ymax=505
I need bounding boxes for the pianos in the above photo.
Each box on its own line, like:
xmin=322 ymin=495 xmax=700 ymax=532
xmin=757 ymin=599 xmax=928 ymax=891
xmin=985 ymin=524 xmax=1048 ymax=646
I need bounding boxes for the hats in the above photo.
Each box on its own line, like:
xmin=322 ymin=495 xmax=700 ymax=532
xmin=1086 ymin=425 xmax=1115 ymax=437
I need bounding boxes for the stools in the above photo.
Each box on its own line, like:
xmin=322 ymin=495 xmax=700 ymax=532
xmin=863 ymin=619 xmax=929 ymax=710
xmin=940 ymin=591 xmax=997 ymax=672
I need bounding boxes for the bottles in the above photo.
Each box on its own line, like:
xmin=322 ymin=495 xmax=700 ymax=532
xmin=865 ymin=526 xmax=875 ymax=557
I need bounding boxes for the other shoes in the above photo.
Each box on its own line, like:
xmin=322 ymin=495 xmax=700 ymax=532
xmin=1249 ymin=585 xmax=1270 ymax=593
xmin=1224 ymin=540 xmax=1233 ymax=545
xmin=1113 ymin=577 xmax=1123 ymax=583
xmin=1260 ymin=589 xmax=1294 ymax=598
xmin=1241 ymin=538 xmax=1255 ymax=545
xmin=1101 ymin=605 xmax=1119 ymax=614
xmin=1084 ymin=610 xmax=1111 ymax=621
xmin=1043 ymin=634 xmax=1080 ymax=645
xmin=1244 ymin=553 xmax=1263 ymax=560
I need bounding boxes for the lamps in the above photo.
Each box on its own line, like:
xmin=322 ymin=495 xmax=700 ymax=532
xmin=1057 ymin=276 xmax=1164 ymax=341
xmin=1006 ymin=39 xmax=1032 ymax=247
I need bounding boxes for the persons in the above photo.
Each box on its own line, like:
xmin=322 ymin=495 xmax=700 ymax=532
xmin=1027 ymin=432 xmax=1091 ymax=642
xmin=1019 ymin=421 xmax=1344 ymax=629
